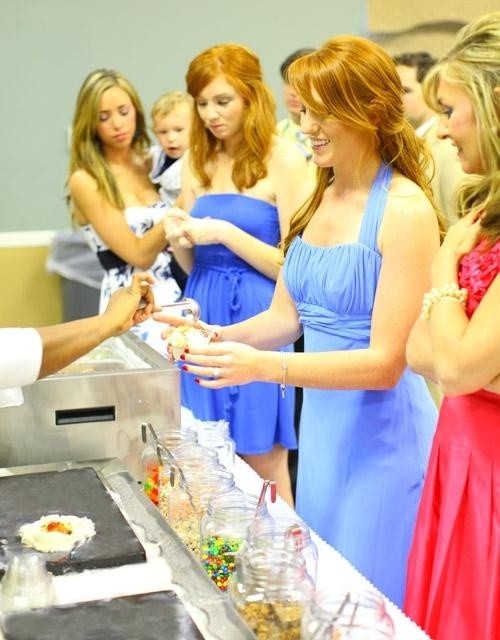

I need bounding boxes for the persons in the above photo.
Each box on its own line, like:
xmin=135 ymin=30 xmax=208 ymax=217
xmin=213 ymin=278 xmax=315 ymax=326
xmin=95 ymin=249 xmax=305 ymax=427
xmin=394 ymin=51 xmax=476 ymax=228
xmin=279 ymin=46 xmax=318 ymax=191
xmin=151 ymin=35 xmax=450 ymax=608
xmin=64 ymin=69 xmax=182 ymax=316
xmin=148 ymin=89 xmax=195 ymax=292
xmin=404 ymin=12 xmax=500 ymax=640
xmin=0 ymin=272 xmax=162 ymax=389
xmin=162 ymin=42 xmax=309 ymax=510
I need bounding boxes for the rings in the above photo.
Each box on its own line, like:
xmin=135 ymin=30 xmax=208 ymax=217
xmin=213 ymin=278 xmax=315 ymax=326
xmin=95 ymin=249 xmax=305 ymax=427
xmin=211 ymin=367 xmax=220 ymax=379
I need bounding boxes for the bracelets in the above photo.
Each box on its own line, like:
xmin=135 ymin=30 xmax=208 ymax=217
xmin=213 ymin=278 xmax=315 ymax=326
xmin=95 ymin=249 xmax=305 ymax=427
xmin=276 ymin=347 xmax=291 ymax=400
xmin=420 ymin=282 xmax=468 ymax=325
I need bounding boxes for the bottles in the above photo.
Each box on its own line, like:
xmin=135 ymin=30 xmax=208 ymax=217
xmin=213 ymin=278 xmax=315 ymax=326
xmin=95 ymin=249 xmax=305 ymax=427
xmin=299 ymin=587 xmax=396 ymax=639
xmin=143 ymin=427 xmax=237 ymax=521
xmin=168 ymin=473 xmax=246 ymax=561
xmin=199 ymin=493 xmax=277 ymax=595
xmin=232 ymin=520 xmax=320 ymax=639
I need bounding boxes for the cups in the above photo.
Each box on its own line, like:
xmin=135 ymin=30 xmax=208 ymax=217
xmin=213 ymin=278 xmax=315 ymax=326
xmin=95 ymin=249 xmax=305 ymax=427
xmin=169 ymin=327 xmax=213 ymax=370
